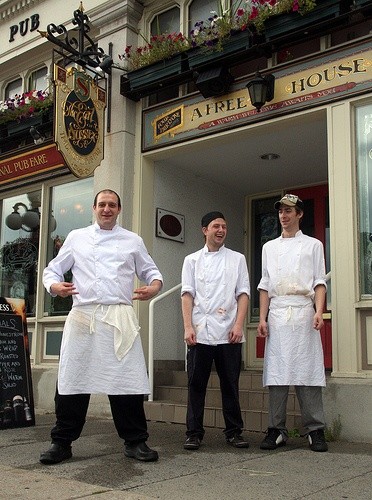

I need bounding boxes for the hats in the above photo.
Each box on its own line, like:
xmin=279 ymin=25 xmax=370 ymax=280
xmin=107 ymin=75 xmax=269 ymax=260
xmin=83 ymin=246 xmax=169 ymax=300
xmin=202 ymin=212 xmax=224 ymax=226
xmin=274 ymin=194 xmax=303 ymax=213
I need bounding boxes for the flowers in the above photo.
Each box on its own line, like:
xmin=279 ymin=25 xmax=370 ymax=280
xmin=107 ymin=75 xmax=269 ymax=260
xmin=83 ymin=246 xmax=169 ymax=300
xmin=0 ymin=89 xmax=54 ymax=123
xmin=118 ymin=0 xmax=318 ymax=71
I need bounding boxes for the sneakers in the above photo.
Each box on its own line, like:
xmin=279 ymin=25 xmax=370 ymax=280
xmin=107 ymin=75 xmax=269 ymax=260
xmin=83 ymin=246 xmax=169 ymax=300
xmin=227 ymin=434 xmax=249 ymax=448
xmin=309 ymin=429 xmax=328 ymax=451
xmin=260 ymin=427 xmax=287 ymax=450
xmin=184 ymin=436 xmax=201 ymax=450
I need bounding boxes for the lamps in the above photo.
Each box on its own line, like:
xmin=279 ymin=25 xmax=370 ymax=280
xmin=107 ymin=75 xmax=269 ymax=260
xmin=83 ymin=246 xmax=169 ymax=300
xmin=245 ymin=62 xmax=275 ymax=113
xmin=196 ymin=67 xmax=234 ymax=98
xmin=30 ymin=125 xmax=45 ymax=145
xmin=99 ymin=56 xmax=113 ymax=72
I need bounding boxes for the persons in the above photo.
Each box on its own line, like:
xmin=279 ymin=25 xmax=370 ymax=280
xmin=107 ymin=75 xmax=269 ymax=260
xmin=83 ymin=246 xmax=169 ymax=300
xmin=257 ymin=194 xmax=328 ymax=452
xmin=181 ymin=212 xmax=251 ymax=449
xmin=40 ymin=189 xmax=164 ymax=460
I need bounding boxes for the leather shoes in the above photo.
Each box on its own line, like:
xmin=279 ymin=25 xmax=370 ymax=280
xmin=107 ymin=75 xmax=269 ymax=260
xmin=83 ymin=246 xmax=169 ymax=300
xmin=124 ymin=442 xmax=158 ymax=461
xmin=39 ymin=444 xmax=72 ymax=464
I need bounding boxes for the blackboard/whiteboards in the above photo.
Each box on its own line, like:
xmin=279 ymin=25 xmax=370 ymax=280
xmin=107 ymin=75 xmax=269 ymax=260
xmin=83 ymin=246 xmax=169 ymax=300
xmin=1 ymin=297 xmax=37 ymax=429
